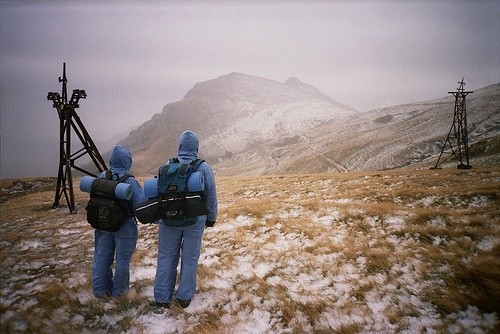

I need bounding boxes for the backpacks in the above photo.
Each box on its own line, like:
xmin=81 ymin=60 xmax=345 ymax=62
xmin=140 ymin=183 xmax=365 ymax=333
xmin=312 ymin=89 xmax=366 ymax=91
xmin=85 ymin=171 xmax=134 ymax=232
xmin=156 ymin=157 xmax=205 ymax=227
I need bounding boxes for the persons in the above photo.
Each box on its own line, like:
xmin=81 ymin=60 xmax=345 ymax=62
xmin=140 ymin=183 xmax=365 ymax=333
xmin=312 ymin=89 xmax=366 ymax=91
xmin=92 ymin=145 xmax=149 ymax=298
xmin=154 ymin=130 xmax=217 ymax=308
